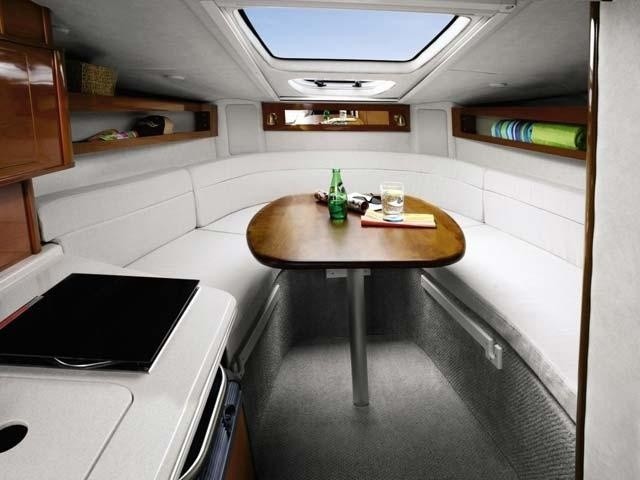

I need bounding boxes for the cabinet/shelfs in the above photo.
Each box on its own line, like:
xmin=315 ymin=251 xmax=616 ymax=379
xmin=0 ymin=0 xmax=76 ymax=273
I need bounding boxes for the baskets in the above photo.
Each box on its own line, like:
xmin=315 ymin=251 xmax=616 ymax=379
xmin=79 ymin=61 xmax=119 ymax=95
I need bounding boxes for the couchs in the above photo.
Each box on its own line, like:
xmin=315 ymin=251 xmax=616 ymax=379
xmin=184 ymin=150 xmax=486 ymax=269
xmin=35 ymin=167 xmax=282 ymax=381
xmin=422 ymin=172 xmax=586 ymax=424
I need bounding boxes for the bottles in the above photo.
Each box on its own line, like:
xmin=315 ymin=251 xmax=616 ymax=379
xmin=327 ymin=168 xmax=348 ymax=221
xmin=322 ymin=110 xmax=330 ymax=122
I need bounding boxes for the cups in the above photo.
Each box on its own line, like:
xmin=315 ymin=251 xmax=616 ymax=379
xmin=339 ymin=110 xmax=346 ymax=120
xmin=381 ymin=182 xmax=404 ymax=222
